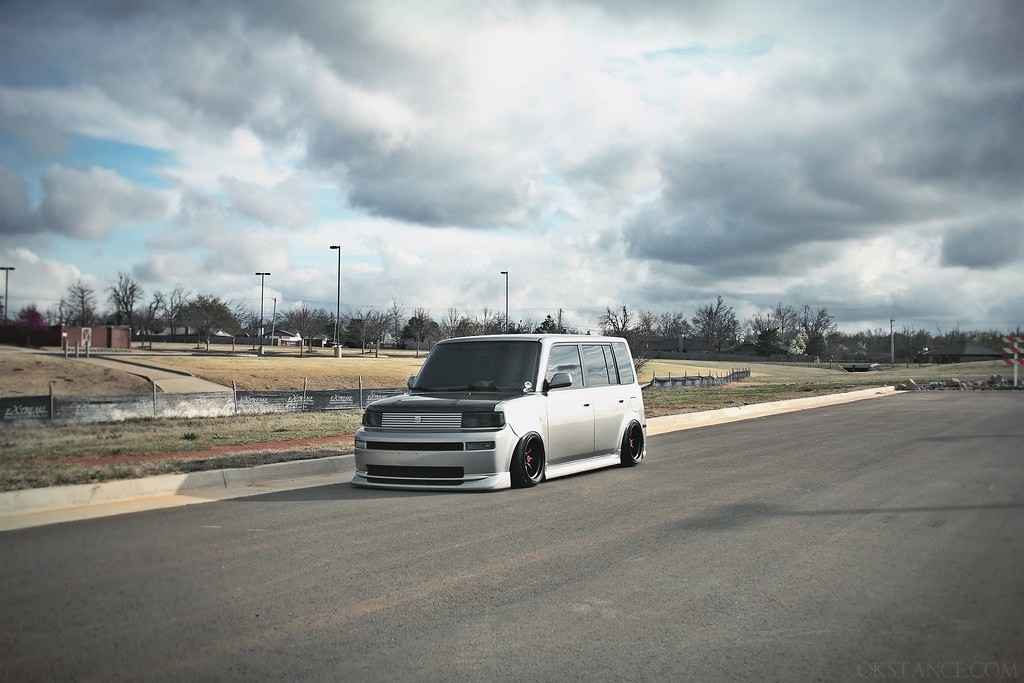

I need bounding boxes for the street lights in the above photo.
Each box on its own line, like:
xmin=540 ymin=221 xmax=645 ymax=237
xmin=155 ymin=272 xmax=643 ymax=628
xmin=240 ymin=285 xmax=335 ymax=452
xmin=255 ymin=272 xmax=270 ymax=348
xmin=0 ymin=266 xmax=15 ymax=324
xmin=330 ymin=245 xmax=343 ymax=348
xmin=500 ymin=270 xmax=509 ymax=333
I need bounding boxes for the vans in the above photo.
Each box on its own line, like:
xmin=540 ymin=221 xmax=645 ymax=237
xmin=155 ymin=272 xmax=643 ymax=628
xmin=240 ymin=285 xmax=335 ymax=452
xmin=351 ymin=333 xmax=648 ymax=492
